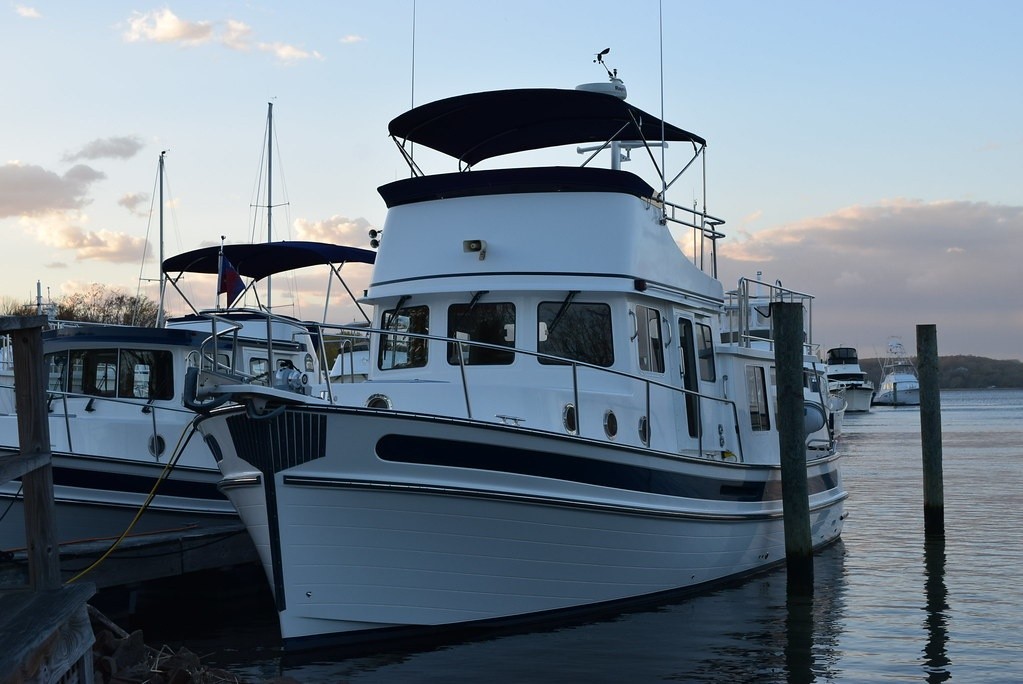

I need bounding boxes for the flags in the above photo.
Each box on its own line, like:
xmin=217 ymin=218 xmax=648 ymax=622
xmin=218 ymin=254 xmax=245 ymax=309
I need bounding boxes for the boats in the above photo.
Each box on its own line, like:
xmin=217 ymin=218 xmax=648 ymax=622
xmin=0 ymin=240 xmax=410 ymax=588
xmin=720 ymin=271 xmax=848 ymax=439
xmin=183 ymin=78 xmax=850 ymax=653
xmin=811 ymin=347 xmax=874 ymax=412
xmin=872 ymin=341 xmax=920 ymax=404
